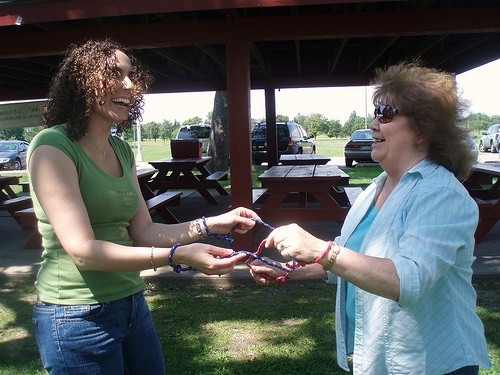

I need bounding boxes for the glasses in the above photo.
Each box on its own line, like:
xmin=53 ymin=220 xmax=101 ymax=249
xmin=374 ymin=105 xmax=408 ymax=124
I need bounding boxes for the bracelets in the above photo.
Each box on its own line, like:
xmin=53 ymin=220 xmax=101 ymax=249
xmin=150 ymin=245 xmax=157 ymax=271
xmin=323 ymin=245 xmax=340 ymax=270
xmin=195 ymin=218 xmax=206 ymax=240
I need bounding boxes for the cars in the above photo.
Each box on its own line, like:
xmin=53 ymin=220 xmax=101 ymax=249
xmin=344 ymin=129 xmax=376 ymax=167
xmin=479 ymin=124 xmax=500 ymax=153
xmin=0 ymin=140 xmax=30 ymax=170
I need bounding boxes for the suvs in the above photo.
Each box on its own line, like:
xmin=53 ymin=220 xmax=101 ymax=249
xmin=175 ymin=123 xmax=211 ymax=153
xmin=251 ymin=121 xmax=316 ymax=165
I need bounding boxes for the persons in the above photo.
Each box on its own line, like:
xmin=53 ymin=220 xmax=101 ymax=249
xmin=250 ymin=63 xmax=491 ymax=375
xmin=26 ymin=41 xmax=260 ymax=375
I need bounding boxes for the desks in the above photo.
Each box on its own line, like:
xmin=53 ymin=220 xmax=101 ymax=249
xmin=24 ymin=169 xmax=181 ymax=249
xmin=148 ymin=157 xmax=230 ymax=206
xmin=279 ymin=154 xmax=331 ymax=165
xmin=253 ymin=165 xmax=351 ymax=235
xmin=0 ymin=176 xmax=34 ymax=230
xmin=472 ymin=163 xmax=500 ymax=244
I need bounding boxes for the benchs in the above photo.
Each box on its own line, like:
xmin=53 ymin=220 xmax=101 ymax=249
xmin=18 ymin=182 xmax=30 ymax=185
xmin=344 ymin=187 xmax=363 ymax=207
xmin=0 ymin=195 xmax=32 ymax=209
xmin=216 ymin=188 xmax=268 ymax=209
xmin=146 ymin=191 xmax=183 ymax=210
xmin=15 ymin=206 xmax=35 ymax=215
xmin=201 ymin=170 xmax=228 ymax=181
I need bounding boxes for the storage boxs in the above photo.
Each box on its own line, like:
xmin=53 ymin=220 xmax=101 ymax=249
xmin=171 ymin=139 xmax=203 ymax=159
